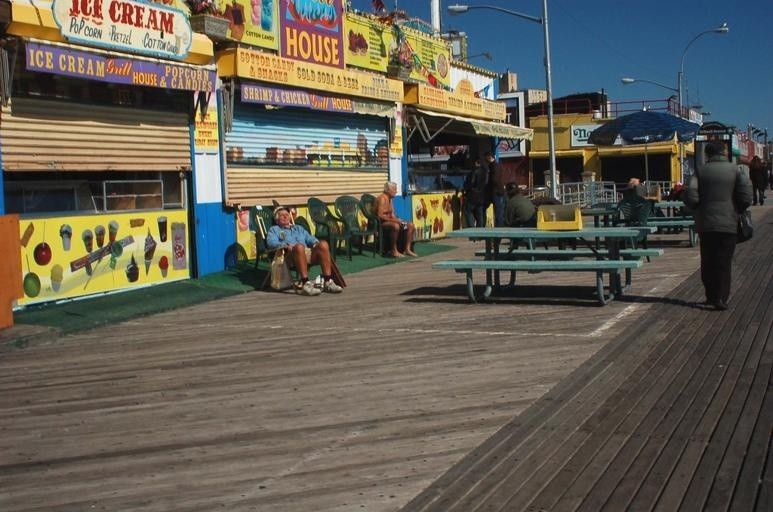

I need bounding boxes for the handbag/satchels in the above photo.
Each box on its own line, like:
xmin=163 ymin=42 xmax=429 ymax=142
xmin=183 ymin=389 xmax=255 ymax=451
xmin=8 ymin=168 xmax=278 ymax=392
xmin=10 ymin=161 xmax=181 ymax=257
xmin=736 ymin=210 xmax=754 ymax=244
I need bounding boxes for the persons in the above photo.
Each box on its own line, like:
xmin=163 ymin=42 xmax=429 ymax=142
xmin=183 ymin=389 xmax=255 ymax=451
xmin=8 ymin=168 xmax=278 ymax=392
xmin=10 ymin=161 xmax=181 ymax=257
xmin=266 ymin=206 xmax=344 ymax=296
xmin=683 ymin=141 xmax=754 ymax=309
xmin=482 ymin=152 xmax=505 ymax=227
xmin=464 ymin=160 xmax=489 ymax=227
xmin=749 ymin=156 xmax=768 ymax=206
xmin=371 ymin=181 xmax=418 ymax=258
xmin=504 ymin=182 xmax=536 ymax=227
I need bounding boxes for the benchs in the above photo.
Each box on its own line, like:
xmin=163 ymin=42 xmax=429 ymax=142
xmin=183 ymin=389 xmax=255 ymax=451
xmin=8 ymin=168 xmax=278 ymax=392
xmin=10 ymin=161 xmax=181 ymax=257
xmin=428 ymin=199 xmax=699 ymax=305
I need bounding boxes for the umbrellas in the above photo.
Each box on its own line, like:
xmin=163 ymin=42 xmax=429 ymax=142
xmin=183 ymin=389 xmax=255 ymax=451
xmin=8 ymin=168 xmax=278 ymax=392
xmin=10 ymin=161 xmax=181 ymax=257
xmin=587 ymin=112 xmax=701 ymax=198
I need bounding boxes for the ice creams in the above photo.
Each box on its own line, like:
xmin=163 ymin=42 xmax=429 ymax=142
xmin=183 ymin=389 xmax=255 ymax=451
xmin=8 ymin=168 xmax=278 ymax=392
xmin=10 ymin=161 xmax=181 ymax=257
xmin=60 ymin=225 xmax=71 ymax=250
xmin=145 ymin=227 xmax=156 ymax=275
xmin=51 ymin=264 xmax=63 ymax=292
xmin=159 ymin=256 xmax=168 ymax=278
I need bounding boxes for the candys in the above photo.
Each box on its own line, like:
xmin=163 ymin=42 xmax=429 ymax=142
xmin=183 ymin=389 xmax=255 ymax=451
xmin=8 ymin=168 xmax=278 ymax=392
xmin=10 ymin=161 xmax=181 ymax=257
xmin=33 ymin=222 xmax=51 ymax=265
xmin=24 ymin=255 xmax=40 ymax=297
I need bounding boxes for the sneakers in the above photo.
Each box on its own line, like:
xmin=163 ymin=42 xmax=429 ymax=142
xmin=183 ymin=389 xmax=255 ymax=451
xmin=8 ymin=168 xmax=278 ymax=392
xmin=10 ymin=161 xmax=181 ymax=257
xmin=323 ymin=278 xmax=343 ymax=293
xmin=714 ymin=297 xmax=729 ymax=310
xmin=302 ymin=280 xmax=322 ymax=296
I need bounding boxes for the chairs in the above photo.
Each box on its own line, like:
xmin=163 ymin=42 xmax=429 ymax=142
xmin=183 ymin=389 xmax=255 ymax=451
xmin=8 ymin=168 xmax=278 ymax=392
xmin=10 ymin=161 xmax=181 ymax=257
xmin=249 ymin=192 xmax=415 ymax=294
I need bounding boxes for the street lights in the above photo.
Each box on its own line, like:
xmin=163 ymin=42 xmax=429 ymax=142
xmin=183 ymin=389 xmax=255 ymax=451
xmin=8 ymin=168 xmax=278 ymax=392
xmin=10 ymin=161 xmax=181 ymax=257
xmin=443 ymin=0 xmax=561 ymax=203
xmin=620 ymin=20 xmax=731 ymax=191
